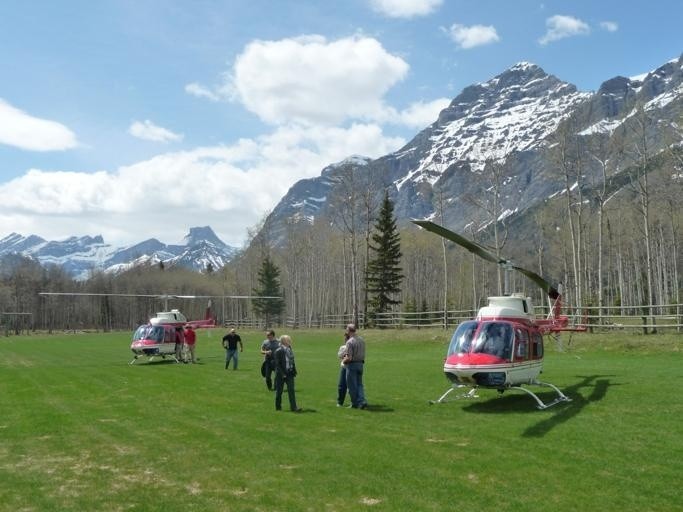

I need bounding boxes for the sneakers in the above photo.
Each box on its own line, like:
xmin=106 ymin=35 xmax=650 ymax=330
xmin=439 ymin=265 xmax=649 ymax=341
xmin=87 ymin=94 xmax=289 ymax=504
xmin=350 ymin=404 xmax=368 ymax=409
xmin=293 ymin=407 xmax=302 ymax=411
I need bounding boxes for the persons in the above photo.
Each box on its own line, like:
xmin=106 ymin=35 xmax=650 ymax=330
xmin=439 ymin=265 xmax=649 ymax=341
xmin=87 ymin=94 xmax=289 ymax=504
xmin=175 ymin=326 xmax=197 ymax=363
xmin=223 ymin=329 xmax=243 ymax=369
xmin=261 ymin=330 xmax=302 ymax=411
xmin=484 ymin=329 xmax=505 ymax=356
xmin=336 ymin=323 xmax=368 ymax=410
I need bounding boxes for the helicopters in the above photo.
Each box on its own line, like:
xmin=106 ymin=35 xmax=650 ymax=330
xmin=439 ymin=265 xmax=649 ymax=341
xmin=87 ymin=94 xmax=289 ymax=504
xmin=37 ymin=291 xmax=282 ymax=366
xmin=412 ymin=220 xmax=588 ymax=409
